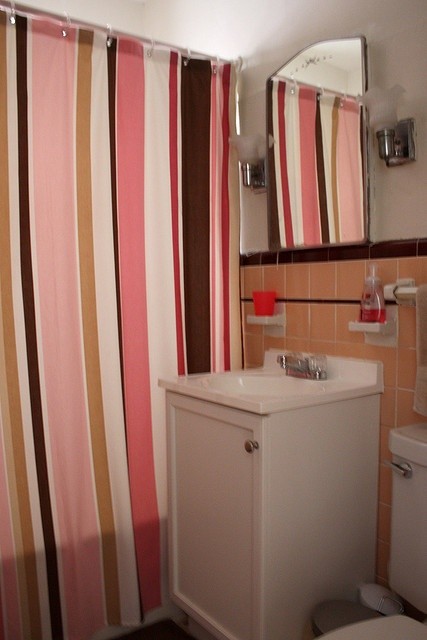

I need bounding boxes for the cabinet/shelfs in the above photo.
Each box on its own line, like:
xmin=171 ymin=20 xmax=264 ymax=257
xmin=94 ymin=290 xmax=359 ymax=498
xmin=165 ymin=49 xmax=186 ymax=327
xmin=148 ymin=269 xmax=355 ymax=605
xmin=166 ymin=390 xmax=382 ymax=635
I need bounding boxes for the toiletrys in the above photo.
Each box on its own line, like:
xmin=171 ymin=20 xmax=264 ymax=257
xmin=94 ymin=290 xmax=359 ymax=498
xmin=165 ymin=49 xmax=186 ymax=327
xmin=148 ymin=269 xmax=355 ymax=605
xmin=360 ymin=263 xmax=386 ymax=322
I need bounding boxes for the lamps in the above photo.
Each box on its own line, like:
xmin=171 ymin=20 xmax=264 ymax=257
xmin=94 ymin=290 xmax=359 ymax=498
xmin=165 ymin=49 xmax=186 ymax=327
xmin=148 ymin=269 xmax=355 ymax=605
xmin=228 ymin=133 xmax=266 ymax=190
xmin=358 ymin=85 xmax=416 ymax=167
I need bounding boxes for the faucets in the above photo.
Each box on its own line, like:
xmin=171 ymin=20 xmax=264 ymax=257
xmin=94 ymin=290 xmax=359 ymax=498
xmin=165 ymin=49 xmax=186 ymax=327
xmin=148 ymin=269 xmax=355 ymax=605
xmin=276 ymin=354 xmax=327 ymax=380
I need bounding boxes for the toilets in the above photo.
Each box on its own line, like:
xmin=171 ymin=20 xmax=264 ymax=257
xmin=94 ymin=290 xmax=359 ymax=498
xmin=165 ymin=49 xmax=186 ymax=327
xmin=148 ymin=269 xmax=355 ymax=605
xmin=313 ymin=422 xmax=427 ymax=640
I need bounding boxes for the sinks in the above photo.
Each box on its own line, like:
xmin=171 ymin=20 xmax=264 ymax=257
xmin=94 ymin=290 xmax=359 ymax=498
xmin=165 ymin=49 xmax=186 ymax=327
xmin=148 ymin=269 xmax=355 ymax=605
xmin=203 ymin=375 xmax=342 ymax=397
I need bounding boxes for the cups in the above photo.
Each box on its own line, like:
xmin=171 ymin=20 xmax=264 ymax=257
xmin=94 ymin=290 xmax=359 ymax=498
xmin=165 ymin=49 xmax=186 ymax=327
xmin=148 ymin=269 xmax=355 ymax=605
xmin=252 ymin=290 xmax=278 ymax=316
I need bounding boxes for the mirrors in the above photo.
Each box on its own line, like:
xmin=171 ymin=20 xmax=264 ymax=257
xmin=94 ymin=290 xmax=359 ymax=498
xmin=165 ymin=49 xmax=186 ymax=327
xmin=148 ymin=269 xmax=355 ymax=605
xmin=262 ymin=33 xmax=374 ymax=252
